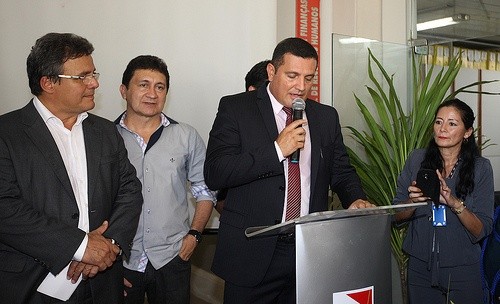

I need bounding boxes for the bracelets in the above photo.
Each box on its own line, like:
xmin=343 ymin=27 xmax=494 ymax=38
xmin=452 ymin=198 xmax=466 ymax=214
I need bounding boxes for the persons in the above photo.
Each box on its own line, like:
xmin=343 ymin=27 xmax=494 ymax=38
xmin=391 ymin=98 xmax=493 ymax=304
xmin=245 ymin=59 xmax=273 ymax=92
xmin=204 ymin=37 xmax=376 ymax=304
xmin=114 ymin=55 xmax=218 ymax=304
xmin=0 ymin=33 xmax=143 ymax=304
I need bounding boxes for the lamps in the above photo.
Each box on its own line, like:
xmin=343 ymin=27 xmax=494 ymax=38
xmin=417 ymin=3 xmax=471 ymax=31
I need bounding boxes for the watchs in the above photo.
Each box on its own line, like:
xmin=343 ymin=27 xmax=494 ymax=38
xmin=188 ymin=229 xmax=203 ymax=243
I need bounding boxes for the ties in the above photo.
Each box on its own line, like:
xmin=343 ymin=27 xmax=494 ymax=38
xmin=282 ymin=105 xmax=301 ymax=221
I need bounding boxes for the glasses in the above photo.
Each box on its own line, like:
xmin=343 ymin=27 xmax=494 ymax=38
xmin=47 ymin=72 xmax=100 ymax=85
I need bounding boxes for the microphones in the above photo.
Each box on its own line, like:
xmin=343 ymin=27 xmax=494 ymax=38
xmin=290 ymin=97 xmax=306 ymax=163
